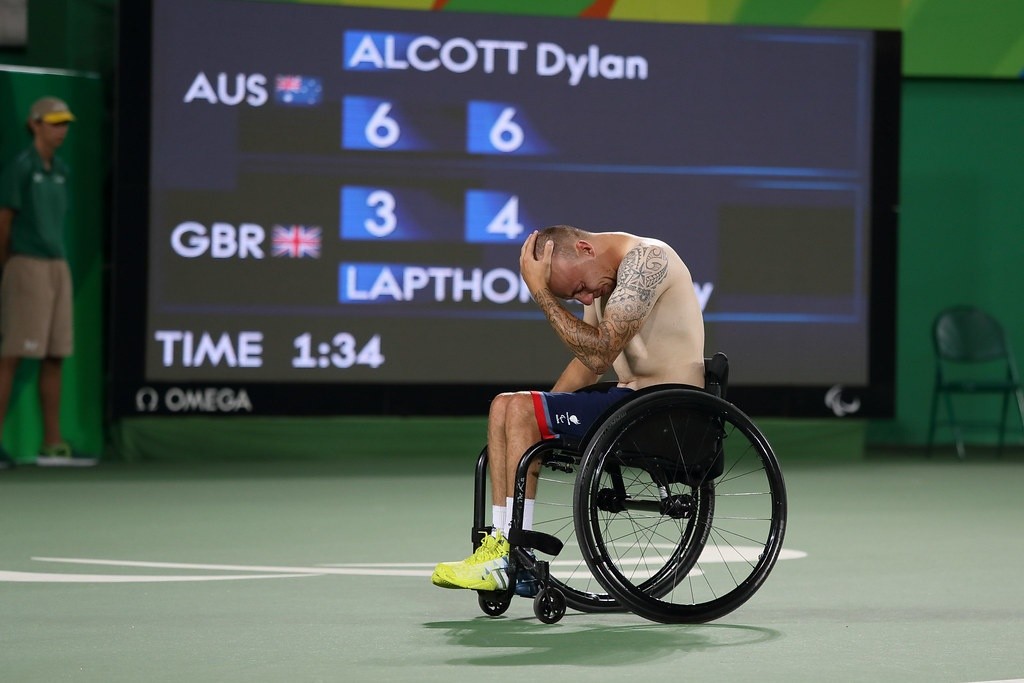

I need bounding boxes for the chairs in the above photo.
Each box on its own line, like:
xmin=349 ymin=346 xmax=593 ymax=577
xmin=925 ymin=306 xmax=1024 ymax=463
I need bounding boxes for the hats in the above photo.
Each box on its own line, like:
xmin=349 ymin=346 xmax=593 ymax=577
xmin=32 ymin=98 xmax=74 ymax=124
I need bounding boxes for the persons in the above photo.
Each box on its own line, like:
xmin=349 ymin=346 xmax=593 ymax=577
xmin=1 ymin=97 xmax=94 ymax=463
xmin=431 ymin=226 xmax=708 ymax=592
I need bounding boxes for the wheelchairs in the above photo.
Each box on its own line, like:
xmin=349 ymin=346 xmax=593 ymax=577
xmin=470 ymin=351 xmax=790 ymax=626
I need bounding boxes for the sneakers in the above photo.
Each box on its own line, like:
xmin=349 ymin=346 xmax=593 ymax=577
xmin=431 ymin=528 xmax=539 ymax=597
xmin=0 ymin=452 xmax=10 ymax=469
xmin=37 ymin=444 xmax=98 ymax=467
